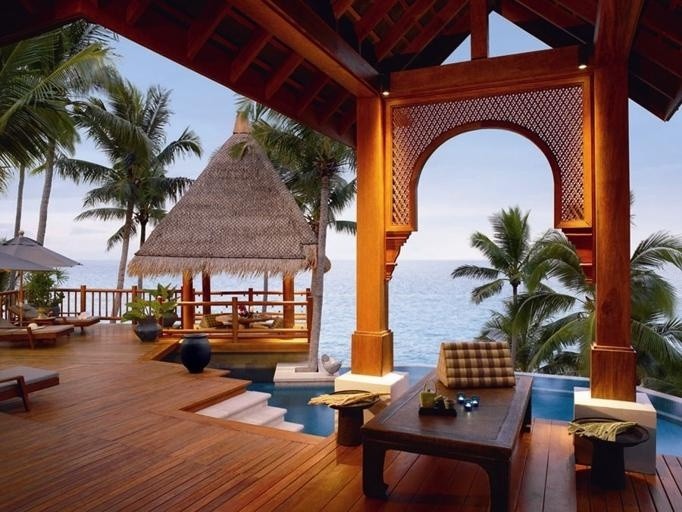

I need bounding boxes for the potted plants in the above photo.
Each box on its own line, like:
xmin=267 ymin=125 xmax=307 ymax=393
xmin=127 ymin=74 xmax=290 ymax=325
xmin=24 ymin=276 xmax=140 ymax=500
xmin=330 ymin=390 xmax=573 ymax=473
xmin=120 ymin=282 xmax=178 ymax=344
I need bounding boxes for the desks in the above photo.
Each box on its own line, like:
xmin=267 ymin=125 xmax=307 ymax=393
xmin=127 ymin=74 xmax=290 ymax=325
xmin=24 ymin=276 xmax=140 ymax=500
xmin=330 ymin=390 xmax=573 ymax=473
xmin=215 ymin=313 xmax=272 ymax=329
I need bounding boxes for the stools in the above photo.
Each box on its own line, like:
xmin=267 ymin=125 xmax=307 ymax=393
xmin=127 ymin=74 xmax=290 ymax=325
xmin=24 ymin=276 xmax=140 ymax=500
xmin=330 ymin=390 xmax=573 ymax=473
xmin=324 ymin=390 xmax=379 ymax=448
xmin=571 ymin=417 xmax=649 ymax=490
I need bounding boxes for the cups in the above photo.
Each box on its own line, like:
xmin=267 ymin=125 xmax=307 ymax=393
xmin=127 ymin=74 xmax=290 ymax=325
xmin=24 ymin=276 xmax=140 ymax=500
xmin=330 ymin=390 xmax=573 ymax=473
xmin=421 ymin=383 xmax=442 ymax=407
xmin=457 ymin=392 xmax=479 ymax=412
xmin=444 ymin=399 xmax=455 ymax=410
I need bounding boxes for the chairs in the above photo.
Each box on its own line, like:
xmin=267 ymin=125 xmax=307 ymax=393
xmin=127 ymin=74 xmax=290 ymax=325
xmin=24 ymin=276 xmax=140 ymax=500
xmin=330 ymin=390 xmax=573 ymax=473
xmin=0 ymin=305 xmax=100 ymax=413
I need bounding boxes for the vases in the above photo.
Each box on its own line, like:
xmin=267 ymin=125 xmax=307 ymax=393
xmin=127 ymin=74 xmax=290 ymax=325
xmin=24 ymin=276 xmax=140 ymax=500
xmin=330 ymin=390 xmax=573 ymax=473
xmin=180 ymin=332 xmax=211 ymax=374
